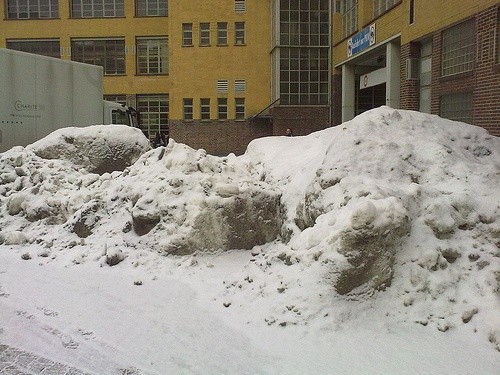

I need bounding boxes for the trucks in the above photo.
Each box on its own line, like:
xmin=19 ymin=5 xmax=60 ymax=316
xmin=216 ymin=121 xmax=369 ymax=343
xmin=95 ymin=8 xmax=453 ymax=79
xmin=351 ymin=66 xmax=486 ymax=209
xmin=0 ymin=48 xmax=142 ymax=153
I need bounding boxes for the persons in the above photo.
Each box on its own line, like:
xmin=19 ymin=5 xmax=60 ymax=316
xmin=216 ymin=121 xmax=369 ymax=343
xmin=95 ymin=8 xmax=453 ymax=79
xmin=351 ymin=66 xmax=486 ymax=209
xmin=152 ymin=132 xmax=167 ymax=147
xmin=285 ymin=128 xmax=294 ymax=138
